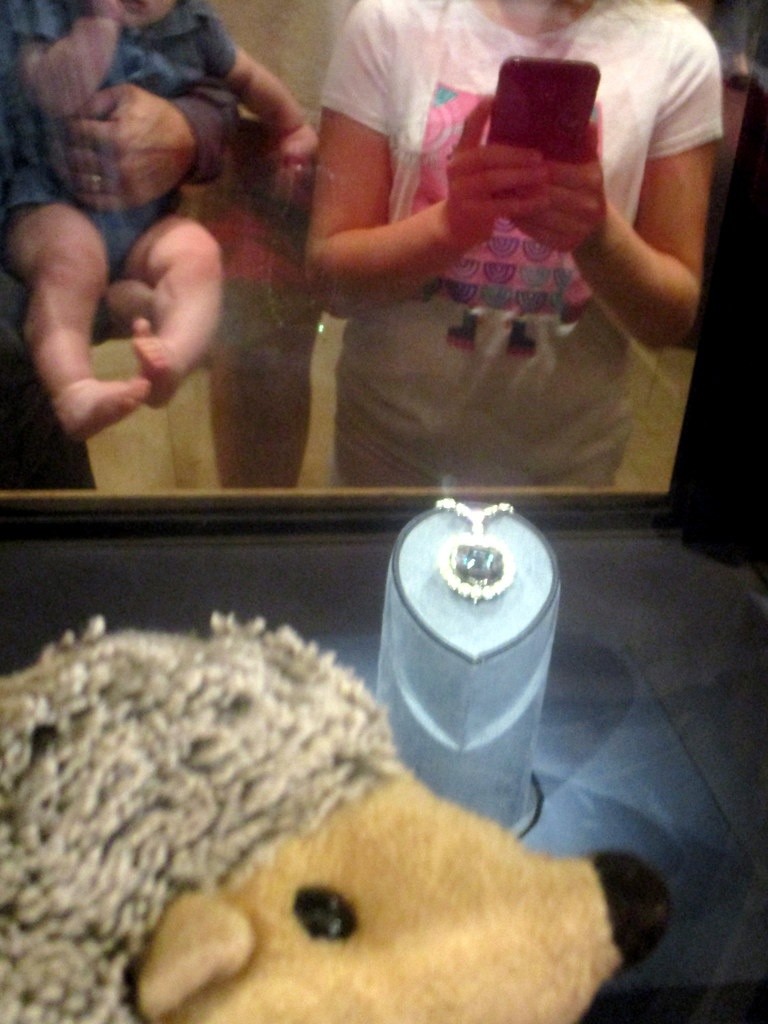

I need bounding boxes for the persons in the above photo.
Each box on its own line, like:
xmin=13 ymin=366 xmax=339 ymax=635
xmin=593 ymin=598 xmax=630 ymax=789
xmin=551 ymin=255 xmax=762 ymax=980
xmin=2 ymin=0 xmax=318 ymax=442
xmin=2 ymin=272 xmax=101 ymax=493
xmin=305 ymin=0 xmax=725 ymax=492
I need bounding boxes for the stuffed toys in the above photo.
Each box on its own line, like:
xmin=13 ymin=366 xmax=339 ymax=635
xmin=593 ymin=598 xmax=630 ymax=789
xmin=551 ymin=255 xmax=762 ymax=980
xmin=0 ymin=609 xmax=673 ymax=1024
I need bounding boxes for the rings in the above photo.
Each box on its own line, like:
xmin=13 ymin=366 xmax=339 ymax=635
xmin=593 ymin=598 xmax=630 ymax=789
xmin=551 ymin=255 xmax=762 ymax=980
xmin=91 ymin=175 xmax=101 ymax=195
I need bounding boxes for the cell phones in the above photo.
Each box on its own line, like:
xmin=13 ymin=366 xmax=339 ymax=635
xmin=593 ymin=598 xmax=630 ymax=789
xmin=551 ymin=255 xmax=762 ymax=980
xmin=488 ymin=56 xmax=602 ymax=163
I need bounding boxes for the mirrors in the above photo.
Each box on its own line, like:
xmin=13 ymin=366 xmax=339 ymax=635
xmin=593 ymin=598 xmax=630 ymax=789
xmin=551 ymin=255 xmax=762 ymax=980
xmin=0 ymin=0 xmax=766 ymax=533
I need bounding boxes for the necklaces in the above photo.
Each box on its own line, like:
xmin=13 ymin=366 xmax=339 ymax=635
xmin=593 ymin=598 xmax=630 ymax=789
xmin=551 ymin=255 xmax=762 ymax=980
xmin=432 ymin=495 xmax=513 ymax=604
